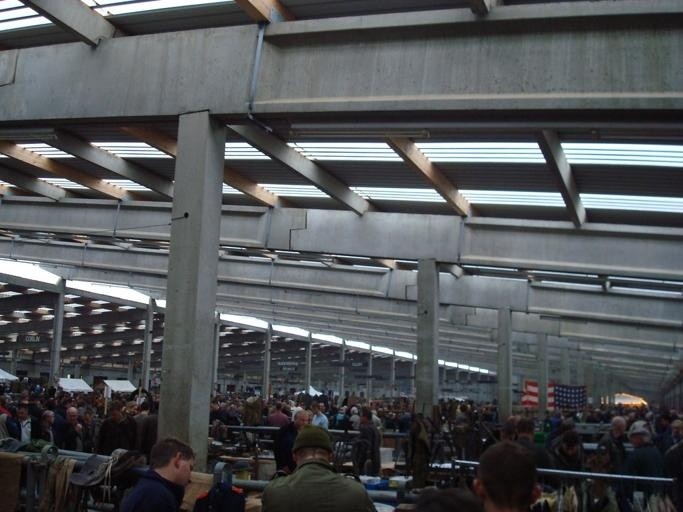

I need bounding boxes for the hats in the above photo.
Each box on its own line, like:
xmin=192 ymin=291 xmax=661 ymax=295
xmin=290 ymin=424 xmax=334 ymax=454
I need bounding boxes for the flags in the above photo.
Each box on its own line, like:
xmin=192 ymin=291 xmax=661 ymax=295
xmin=522 ymin=383 xmax=586 ymax=407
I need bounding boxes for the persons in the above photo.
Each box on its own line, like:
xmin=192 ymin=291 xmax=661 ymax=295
xmin=120 ymin=437 xmax=195 ymax=511
xmin=209 ymin=386 xmax=383 ymax=478
xmin=261 ymin=424 xmax=376 ymax=510
xmin=2 ymin=382 xmax=158 ymax=466
xmin=377 ymin=394 xmax=682 ymax=510
